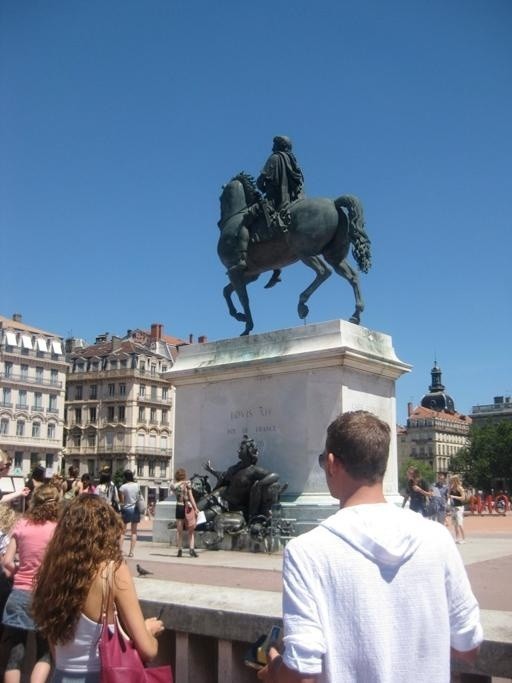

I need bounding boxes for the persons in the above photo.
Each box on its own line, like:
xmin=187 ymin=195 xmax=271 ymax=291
xmin=0 ymin=450 xmax=166 ymax=682
xmin=168 ymin=432 xmax=280 ymax=533
xmin=224 ymin=134 xmax=304 ymax=289
xmin=446 ymin=474 xmax=468 ymax=544
xmin=427 ymin=470 xmax=449 ymax=524
xmin=169 ymin=467 xmax=206 ymax=558
xmin=401 ymin=465 xmax=435 ymax=519
xmin=241 ymin=410 xmax=483 ymax=683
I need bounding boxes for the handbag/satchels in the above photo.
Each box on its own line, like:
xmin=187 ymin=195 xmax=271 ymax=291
xmin=137 ymin=494 xmax=146 ymax=513
xmin=185 ymin=501 xmax=196 ymax=531
xmin=98 ymin=612 xmax=173 ymax=683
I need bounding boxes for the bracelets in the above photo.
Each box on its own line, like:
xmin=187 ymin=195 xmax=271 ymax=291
xmin=420 ymin=489 xmax=424 ymax=494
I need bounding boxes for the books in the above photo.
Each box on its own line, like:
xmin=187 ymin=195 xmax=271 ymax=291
xmin=256 ymin=624 xmax=284 ymax=663
xmin=243 ymin=632 xmax=266 ymax=670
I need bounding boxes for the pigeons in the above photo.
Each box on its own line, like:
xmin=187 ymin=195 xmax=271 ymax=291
xmin=137 ymin=564 xmax=153 ymax=577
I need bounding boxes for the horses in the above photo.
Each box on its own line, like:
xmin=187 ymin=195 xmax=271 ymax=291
xmin=217 ymin=171 xmax=372 ymax=337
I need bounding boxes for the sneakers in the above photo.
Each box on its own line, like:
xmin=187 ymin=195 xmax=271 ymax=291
xmin=177 ymin=550 xmax=182 ymax=557
xmin=190 ymin=549 xmax=197 ymax=557
xmin=455 ymin=537 xmax=467 ymax=544
xmin=129 ymin=552 xmax=134 ymax=557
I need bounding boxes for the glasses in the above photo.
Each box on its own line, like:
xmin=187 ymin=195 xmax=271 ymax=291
xmin=319 ymin=451 xmax=326 ymax=469
